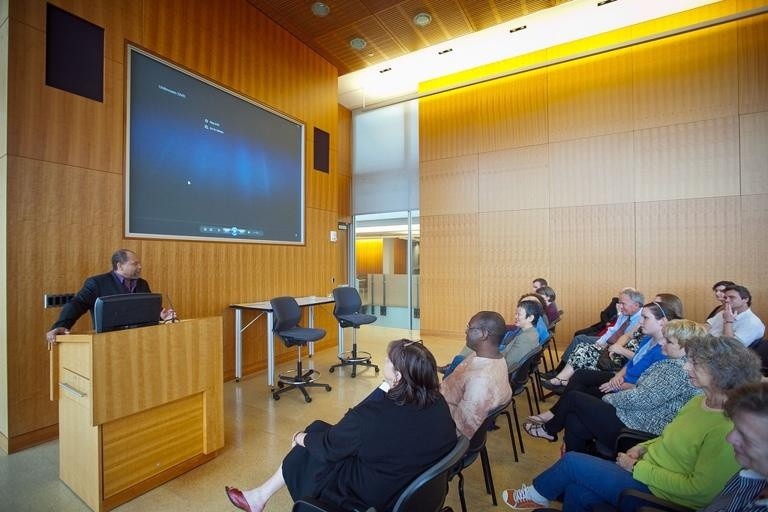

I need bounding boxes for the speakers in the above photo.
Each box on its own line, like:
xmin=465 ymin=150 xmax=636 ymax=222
xmin=46 ymin=2 xmax=105 ymax=104
xmin=314 ymin=127 xmax=330 ymax=174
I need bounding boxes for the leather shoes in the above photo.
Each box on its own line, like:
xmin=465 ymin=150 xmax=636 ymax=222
xmin=226 ymin=486 xmax=262 ymax=512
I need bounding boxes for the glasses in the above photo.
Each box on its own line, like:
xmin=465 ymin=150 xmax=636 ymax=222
xmin=466 ymin=321 xmax=486 ymax=331
xmin=402 ymin=338 xmax=423 ymax=361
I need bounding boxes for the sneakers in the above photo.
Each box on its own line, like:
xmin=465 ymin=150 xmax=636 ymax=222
xmin=501 ymin=488 xmax=551 ymax=510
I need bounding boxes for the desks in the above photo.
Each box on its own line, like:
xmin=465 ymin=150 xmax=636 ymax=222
xmin=50 ymin=317 xmax=224 ymax=512
xmin=228 ymin=296 xmax=344 ymax=393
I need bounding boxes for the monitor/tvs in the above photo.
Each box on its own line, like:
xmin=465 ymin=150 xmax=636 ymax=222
xmin=95 ymin=293 xmax=163 ymax=333
xmin=126 ymin=44 xmax=305 ymax=244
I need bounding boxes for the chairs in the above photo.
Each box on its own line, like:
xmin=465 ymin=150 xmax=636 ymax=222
xmin=329 ymin=287 xmax=379 ymax=378
xmin=542 ymin=310 xmax=563 ymax=371
xmin=533 ymin=331 xmax=553 ymax=402
xmin=501 ymin=361 xmax=525 ymax=463
xmin=290 ymin=434 xmax=470 ymax=512
xmin=458 ymin=398 xmax=512 ymax=512
xmin=270 ymin=296 xmax=331 ymax=402
xmin=512 ymin=346 xmax=543 ymax=416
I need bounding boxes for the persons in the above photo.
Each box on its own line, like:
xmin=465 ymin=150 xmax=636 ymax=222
xmin=503 ymin=334 xmax=762 ymax=512
xmin=540 ymin=287 xmax=643 ymax=380
xmin=705 ymin=285 xmax=765 ymax=348
xmin=525 ymin=302 xmax=684 ymax=424
xmin=706 ymin=281 xmax=734 ymax=320
xmin=540 ymin=293 xmax=683 ymax=394
xmin=436 ymin=294 xmax=549 ymax=374
xmin=701 ymin=382 xmax=768 ymax=512
xmin=523 ymin=319 xmax=707 ymax=451
xmin=530 ymin=279 xmax=549 ymax=292
xmin=224 ymin=339 xmax=458 ymax=512
xmin=439 ymin=311 xmax=512 ymax=441
xmin=502 ymin=287 xmax=557 ymax=333
xmin=573 ymin=297 xmax=620 ymax=337
xmin=46 ymin=249 xmax=177 ymax=345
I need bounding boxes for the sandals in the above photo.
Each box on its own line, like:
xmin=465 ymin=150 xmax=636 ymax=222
xmin=543 ymin=377 xmax=569 ymax=392
xmin=607 ymin=319 xmax=630 ymax=345
xmin=523 ymin=422 xmax=558 ymax=443
xmin=527 ymin=413 xmax=549 ymax=423
